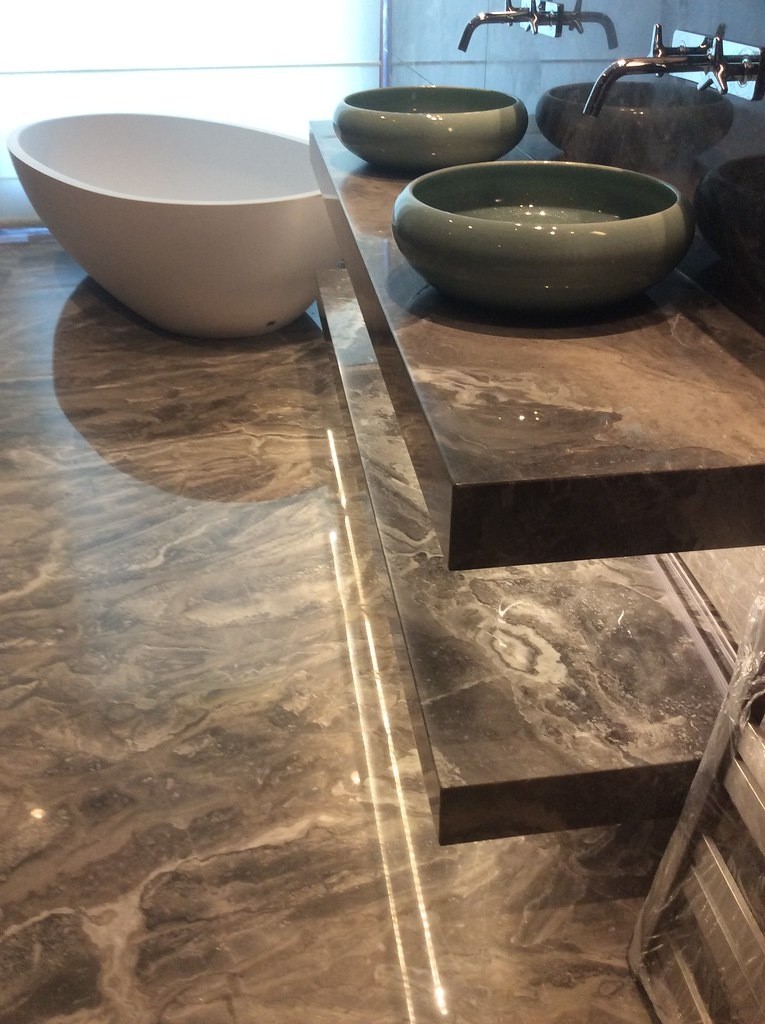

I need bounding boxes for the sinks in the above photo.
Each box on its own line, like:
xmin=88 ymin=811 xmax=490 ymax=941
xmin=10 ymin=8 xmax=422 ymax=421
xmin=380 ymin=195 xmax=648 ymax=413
xmin=388 ymin=161 xmax=696 ymax=314
xmin=695 ymin=155 xmax=764 ymax=266
xmin=534 ymin=80 xmax=734 ymax=165
xmin=332 ymin=86 xmax=530 ymax=170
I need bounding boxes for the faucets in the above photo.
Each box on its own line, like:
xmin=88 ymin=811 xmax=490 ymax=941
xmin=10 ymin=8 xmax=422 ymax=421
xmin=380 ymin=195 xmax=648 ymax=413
xmin=563 ymin=9 xmax=619 ymax=50
xmin=583 ymin=56 xmax=713 ymax=118
xmin=713 ymin=51 xmax=763 ymax=82
xmin=457 ymin=10 xmax=530 ymax=53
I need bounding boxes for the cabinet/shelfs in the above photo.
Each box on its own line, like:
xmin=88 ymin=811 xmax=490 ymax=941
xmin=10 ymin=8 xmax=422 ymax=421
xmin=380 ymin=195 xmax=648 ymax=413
xmin=310 ymin=117 xmax=765 ymax=846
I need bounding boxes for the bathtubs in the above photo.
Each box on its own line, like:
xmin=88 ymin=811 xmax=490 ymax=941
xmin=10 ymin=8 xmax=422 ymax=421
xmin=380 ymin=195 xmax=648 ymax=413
xmin=9 ymin=112 xmax=338 ymax=338
